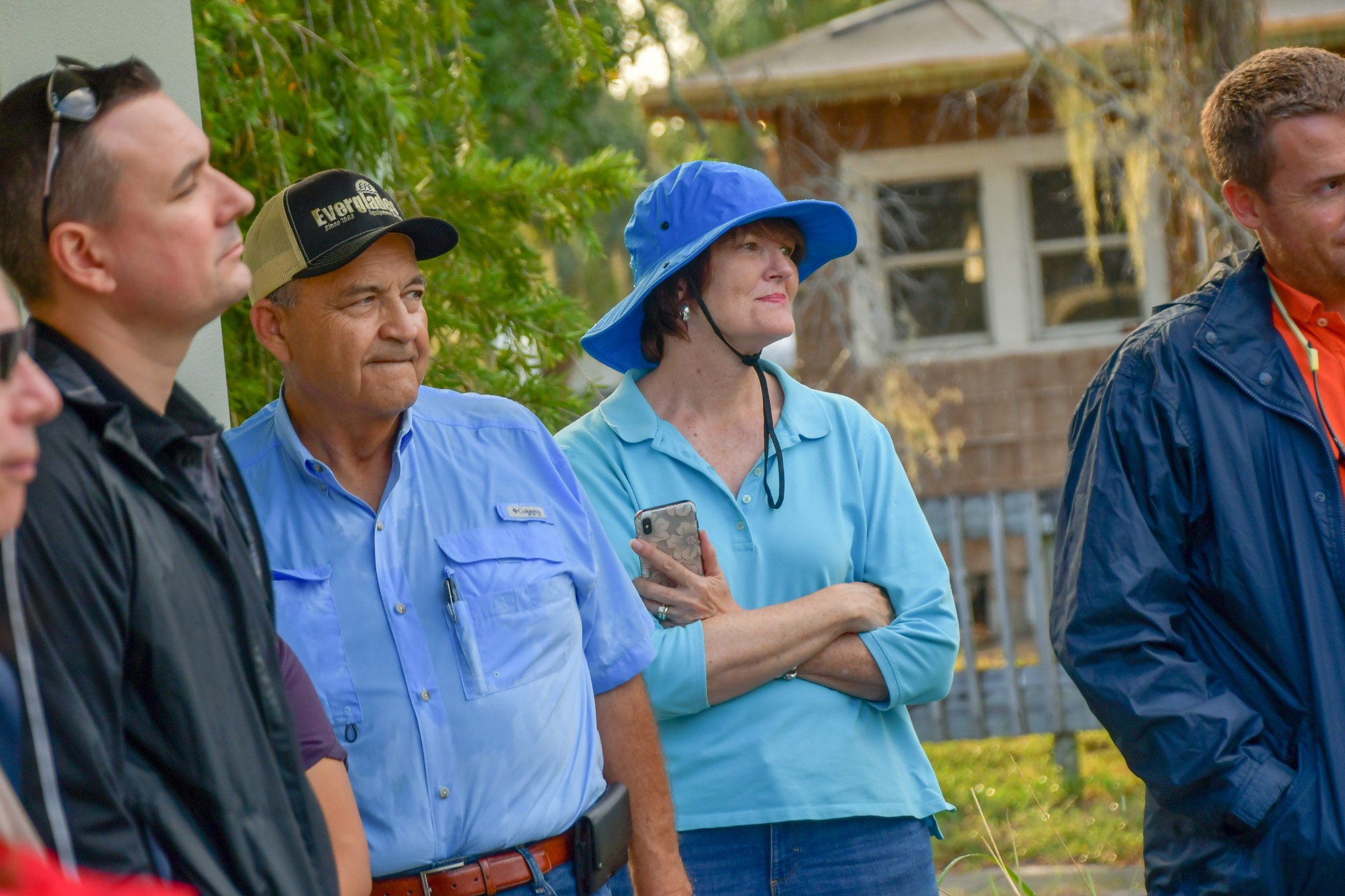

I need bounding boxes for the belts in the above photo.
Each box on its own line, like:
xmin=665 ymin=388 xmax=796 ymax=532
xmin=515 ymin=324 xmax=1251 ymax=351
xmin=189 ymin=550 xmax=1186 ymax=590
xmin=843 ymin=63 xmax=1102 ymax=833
xmin=371 ymin=828 xmax=576 ymax=896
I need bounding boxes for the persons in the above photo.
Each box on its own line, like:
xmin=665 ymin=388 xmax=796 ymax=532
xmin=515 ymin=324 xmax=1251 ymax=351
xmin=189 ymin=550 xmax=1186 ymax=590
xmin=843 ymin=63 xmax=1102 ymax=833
xmin=1053 ymin=44 xmax=1345 ymax=895
xmin=273 ymin=628 xmax=377 ymax=896
xmin=226 ymin=165 xmax=700 ymax=896
xmin=0 ymin=51 xmax=346 ymax=896
xmin=0 ymin=265 xmax=201 ymax=896
xmin=549 ymin=153 xmax=968 ymax=896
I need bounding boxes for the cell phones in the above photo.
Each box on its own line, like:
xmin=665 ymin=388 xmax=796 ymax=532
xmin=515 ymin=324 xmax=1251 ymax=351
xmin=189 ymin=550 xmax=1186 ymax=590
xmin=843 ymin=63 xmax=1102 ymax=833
xmin=635 ymin=499 xmax=704 ymax=631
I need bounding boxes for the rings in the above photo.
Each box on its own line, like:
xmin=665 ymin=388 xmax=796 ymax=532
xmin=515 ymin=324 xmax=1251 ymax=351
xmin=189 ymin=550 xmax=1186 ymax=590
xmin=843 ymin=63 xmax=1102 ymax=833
xmin=658 ymin=603 xmax=666 ymax=621
xmin=662 ymin=605 xmax=669 ymax=621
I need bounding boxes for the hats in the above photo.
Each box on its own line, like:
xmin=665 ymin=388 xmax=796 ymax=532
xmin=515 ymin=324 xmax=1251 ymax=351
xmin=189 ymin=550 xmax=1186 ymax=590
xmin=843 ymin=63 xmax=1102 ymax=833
xmin=579 ymin=158 xmax=857 ymax=375
xmin=242 ymin=168 xmax=460 ymax=310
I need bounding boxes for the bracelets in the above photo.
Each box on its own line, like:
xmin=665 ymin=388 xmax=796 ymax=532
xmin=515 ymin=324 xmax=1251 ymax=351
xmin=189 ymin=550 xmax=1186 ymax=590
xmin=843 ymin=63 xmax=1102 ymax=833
xmin=783 ymin=666 xmax=798 ymax=681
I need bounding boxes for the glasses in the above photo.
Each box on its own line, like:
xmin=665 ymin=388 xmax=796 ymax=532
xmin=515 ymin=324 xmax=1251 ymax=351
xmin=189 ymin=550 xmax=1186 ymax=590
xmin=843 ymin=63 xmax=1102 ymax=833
xmin=0 ymin=317 xmax=44 ymax=381
xmin=34 ymin=55 xmax=104 ymax=245
